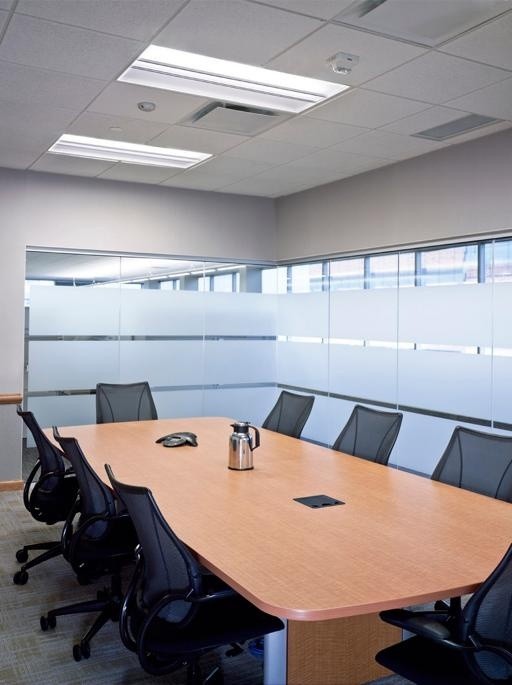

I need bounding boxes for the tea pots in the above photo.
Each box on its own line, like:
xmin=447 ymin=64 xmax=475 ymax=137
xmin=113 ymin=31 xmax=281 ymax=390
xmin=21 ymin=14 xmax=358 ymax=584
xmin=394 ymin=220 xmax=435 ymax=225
xmin=227 ymin=421 xmax=260 ymax=472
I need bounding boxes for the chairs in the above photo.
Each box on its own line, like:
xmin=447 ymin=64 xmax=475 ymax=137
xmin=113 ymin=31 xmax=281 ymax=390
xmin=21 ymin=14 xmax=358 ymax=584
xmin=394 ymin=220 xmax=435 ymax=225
xmin=96 ymin=382 xmax=158 ymax=424
xmin=431 ymin=425 xmax=510 ymax=505
xmin=40 ymin=426 xmax=141 ymax=662
xmin=263 ymin=390 xmax=315 ymax=439
xmin=105 ymin=464 xmax=285 ymax=684
xmin=330 ymin=405 xmax=403 ymax=466
xmin=375 ymin=543 xmax=511 ymax=684
xmin=14 ymin=406 xmax=85 ymax=585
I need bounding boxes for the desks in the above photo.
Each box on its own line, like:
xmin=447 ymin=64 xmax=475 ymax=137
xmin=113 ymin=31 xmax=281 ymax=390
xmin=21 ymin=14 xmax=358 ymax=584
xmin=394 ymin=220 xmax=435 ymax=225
xmin=41 ymin=416 xmax=511 ymax=682
